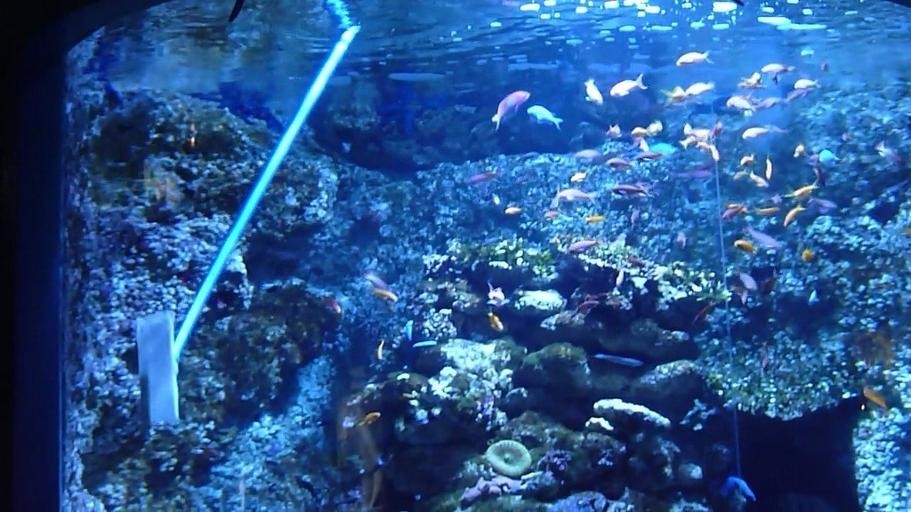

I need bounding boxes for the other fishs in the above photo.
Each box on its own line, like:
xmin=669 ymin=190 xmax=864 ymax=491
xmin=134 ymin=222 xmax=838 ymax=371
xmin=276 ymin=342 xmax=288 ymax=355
xmin=463 ymin=46 xmax=843 ymax=332
